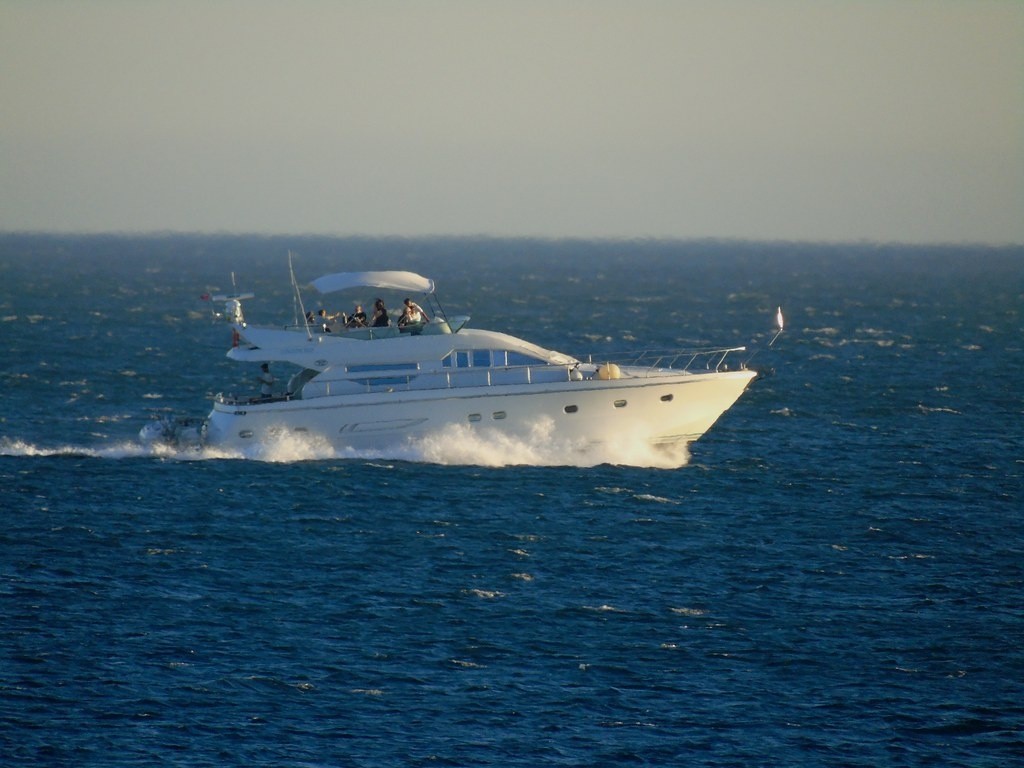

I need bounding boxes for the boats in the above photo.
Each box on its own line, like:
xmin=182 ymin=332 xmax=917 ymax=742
xmin=199 ymin=272 xmax=760 ymax=467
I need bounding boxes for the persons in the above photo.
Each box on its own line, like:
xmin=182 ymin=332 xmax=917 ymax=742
xmin=373 ymin=299 xmax=392 ymax=327
xmin=306 ymin=311 xmax=316 ymax=325
xmin=319 ymin=309 xmax=340 ymax=323
xmin=349 ymin=305 xmax=367 ymax=329
xmin=398 ymin=298 xmax=430 ymax=326
